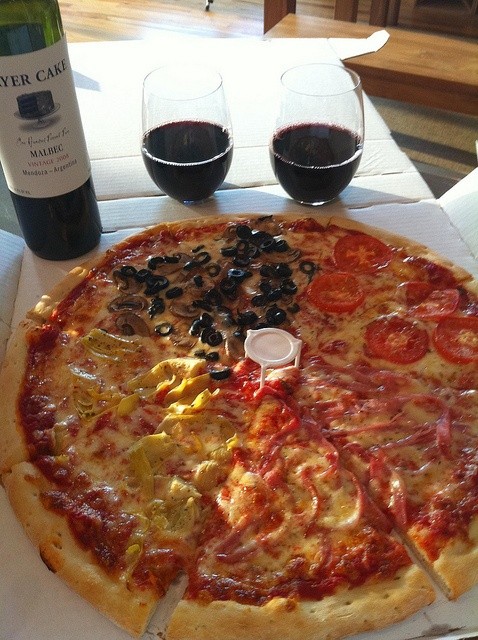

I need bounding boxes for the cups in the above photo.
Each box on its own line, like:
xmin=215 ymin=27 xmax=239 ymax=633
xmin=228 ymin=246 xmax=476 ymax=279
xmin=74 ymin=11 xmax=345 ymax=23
xmin=269 ymin=62 xmax=366 ymax=207
xmin=140 ymin=64 xmax=233 ymax=203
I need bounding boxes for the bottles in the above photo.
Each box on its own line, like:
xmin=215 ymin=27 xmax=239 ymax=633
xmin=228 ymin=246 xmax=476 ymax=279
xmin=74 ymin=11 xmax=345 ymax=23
xmin=0 ymin=0 xmax=102 ymax=259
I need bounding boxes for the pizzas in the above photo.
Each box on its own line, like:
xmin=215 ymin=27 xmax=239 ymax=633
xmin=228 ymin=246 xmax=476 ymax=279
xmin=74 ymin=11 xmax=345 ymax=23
xmin=0 ymin=212 xmax=476 ymax=640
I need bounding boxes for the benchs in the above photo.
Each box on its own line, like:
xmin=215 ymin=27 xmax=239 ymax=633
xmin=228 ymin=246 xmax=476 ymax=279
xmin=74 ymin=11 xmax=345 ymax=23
xmin=263 ymin=14 xmax=478 ymax=117
